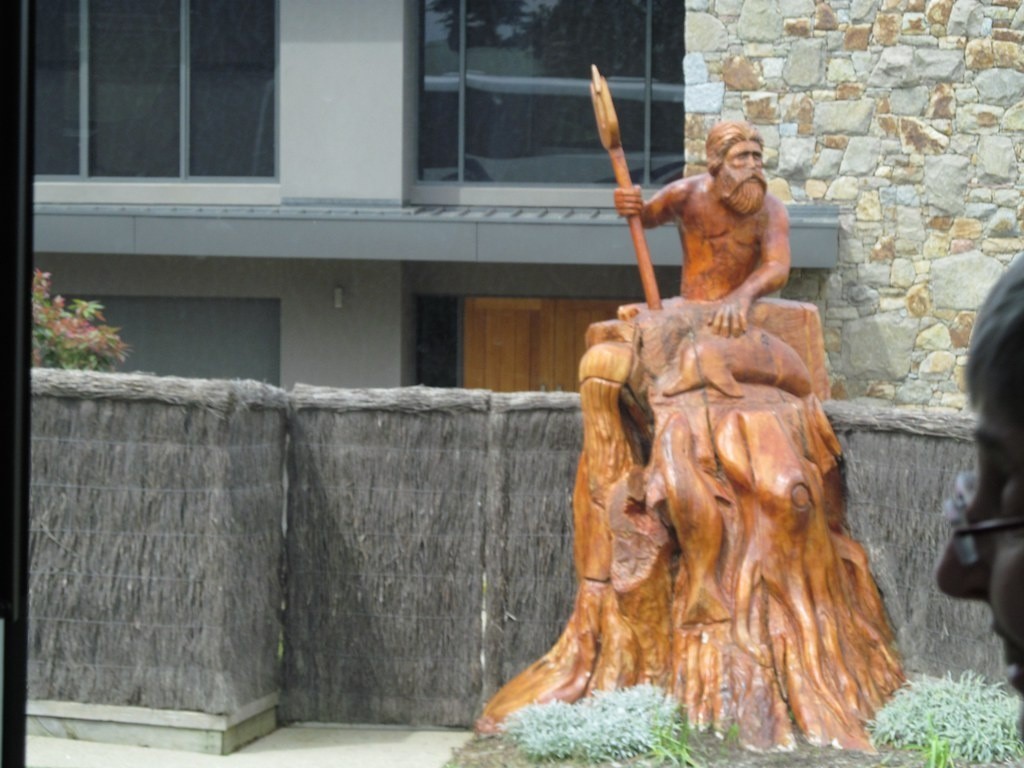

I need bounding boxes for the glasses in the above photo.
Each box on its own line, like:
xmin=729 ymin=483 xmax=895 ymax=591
xmin=939 ymin=465 xmax=1024 ymax=565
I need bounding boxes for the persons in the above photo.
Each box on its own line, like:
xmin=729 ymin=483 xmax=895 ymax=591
xmin=938 ymin=252 xmax=1024 ymax=746
xmin=613 ymin=122 xmax=790 ymax=332
